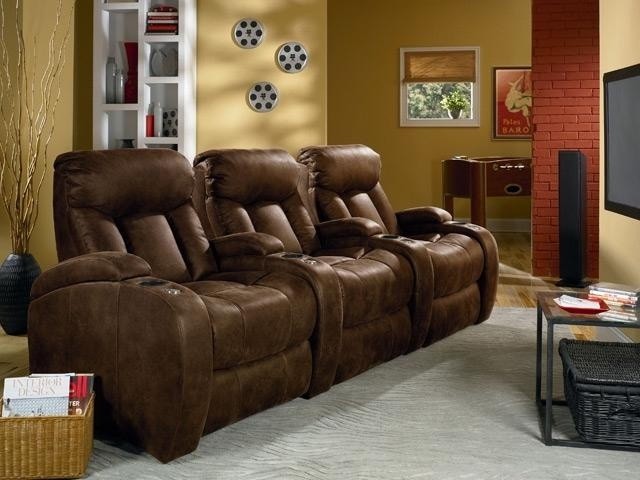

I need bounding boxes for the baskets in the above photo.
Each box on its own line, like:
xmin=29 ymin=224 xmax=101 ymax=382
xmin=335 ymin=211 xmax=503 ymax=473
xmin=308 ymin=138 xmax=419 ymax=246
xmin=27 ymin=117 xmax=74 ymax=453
xmin=560 ymin=338 xmax=639 ymax=451
xmin=0 ymin=391 xmax=95 ymax=479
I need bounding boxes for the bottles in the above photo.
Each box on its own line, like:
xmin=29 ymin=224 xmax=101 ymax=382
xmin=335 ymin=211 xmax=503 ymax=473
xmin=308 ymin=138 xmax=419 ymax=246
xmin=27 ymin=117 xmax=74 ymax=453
xmin=154 ymin=102 xmax=163 ymax=136
xmin=115 ymin=69 xmax=126 ymax=102
xmin=106 ymin=57 xmax=117 ymax=102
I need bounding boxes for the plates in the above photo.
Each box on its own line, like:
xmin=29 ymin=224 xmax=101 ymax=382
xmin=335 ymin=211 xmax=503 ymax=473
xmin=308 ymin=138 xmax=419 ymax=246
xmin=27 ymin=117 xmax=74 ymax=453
xmin=152 ymin=47 xmax=179 ymax=76
xmin=561 ymin=297 xmax=611 ymax=314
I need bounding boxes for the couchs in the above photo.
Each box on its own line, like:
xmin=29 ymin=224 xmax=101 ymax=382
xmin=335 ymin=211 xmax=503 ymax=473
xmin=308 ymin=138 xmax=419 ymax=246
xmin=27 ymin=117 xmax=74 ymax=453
xmin=295 ymin=144 xmax=499 ymax=348
xmin=192 ymin=152 xmax=414 ymax=401
xmin=53 ymin=150 xmax=313 ymax=466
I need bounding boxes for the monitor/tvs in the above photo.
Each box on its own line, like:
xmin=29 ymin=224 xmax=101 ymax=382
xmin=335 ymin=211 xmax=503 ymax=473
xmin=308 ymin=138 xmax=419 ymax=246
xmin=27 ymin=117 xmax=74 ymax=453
xmin=603 ymin=63 xmax=640 ymax=221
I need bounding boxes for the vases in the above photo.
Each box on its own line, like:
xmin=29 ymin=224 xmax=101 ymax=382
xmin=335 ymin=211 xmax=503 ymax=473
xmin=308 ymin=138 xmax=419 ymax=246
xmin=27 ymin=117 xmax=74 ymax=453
xmin=1 ymin=254 xmax=42 ymax=336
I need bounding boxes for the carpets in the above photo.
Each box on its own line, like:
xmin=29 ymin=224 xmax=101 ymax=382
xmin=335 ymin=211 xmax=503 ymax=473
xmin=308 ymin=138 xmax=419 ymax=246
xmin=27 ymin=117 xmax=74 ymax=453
xmin=83 ymin=300 xmax=640 ymax=479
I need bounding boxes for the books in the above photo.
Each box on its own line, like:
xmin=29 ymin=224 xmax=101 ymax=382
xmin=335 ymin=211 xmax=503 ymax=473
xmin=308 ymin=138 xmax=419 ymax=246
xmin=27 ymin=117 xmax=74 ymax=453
xmin=1 ymin=376 xmax=70 ymax=418
xmin=588 ymin=282 xmax=638 ymax=324
xmin=31 ymin=373 xmax=95 ymax=416
xmin=143 ymin=5 xmax=178 ymax=35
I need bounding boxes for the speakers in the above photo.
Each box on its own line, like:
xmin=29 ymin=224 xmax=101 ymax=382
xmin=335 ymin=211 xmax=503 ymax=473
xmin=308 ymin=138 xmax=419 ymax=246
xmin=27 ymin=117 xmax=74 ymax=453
xmin=555 ymin=151 xmax=591 ymax=288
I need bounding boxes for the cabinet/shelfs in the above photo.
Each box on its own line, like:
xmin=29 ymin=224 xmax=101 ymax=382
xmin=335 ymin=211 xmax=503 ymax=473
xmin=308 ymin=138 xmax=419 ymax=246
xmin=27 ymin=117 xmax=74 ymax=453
xmin=438 ymin=153 xmax=531 ymax=229
xmin=90 ymin=1 xmax=199 ymax=172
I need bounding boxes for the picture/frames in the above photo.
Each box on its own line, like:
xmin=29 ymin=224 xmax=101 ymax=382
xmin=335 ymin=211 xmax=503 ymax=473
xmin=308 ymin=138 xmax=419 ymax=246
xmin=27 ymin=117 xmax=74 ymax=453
xmin=491 ymin=65 xmax=537 ymax=141
